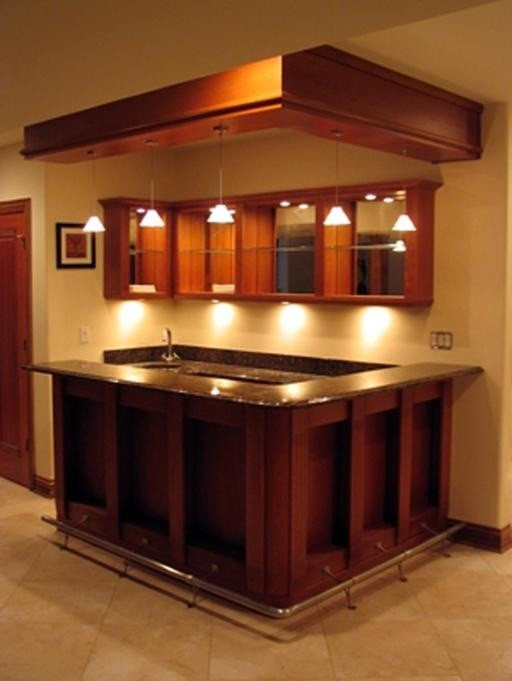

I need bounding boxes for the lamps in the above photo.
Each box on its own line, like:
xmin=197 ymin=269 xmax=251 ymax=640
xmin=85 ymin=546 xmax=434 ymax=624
xmin=206 ymin=123 xmax=231 ymax=222
xmin=136 ymin=135 xmax=168 ymax=230
xmin=388 ymin=148 xmax=420 ymax=234
xmin=321 ymin=125 xmax=350 ymax=230
xmin=83 ymin=148 xmax=107 ymax=236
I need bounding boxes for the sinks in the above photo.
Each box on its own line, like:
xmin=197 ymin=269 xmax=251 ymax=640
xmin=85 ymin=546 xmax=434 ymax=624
xmin=140 ymin=364 xmax=180 ymax=369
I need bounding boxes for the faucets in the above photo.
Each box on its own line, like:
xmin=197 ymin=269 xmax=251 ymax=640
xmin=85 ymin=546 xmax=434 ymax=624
xmin=160 ymin=326 xmax=176 ymax=362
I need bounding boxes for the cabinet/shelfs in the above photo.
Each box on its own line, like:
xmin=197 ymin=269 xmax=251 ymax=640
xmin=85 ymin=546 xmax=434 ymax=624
xmin=108 ymin=190 xmax=433 ymax=306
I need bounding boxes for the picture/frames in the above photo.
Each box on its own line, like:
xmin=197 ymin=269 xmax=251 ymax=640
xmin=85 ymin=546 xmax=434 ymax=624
xmin=56 ymin=222 xmax=97 ymax=270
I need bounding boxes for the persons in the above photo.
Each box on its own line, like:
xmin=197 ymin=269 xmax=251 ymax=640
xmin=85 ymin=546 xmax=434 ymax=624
xmin=358 ymin=261 xmax=368 ymax=295
